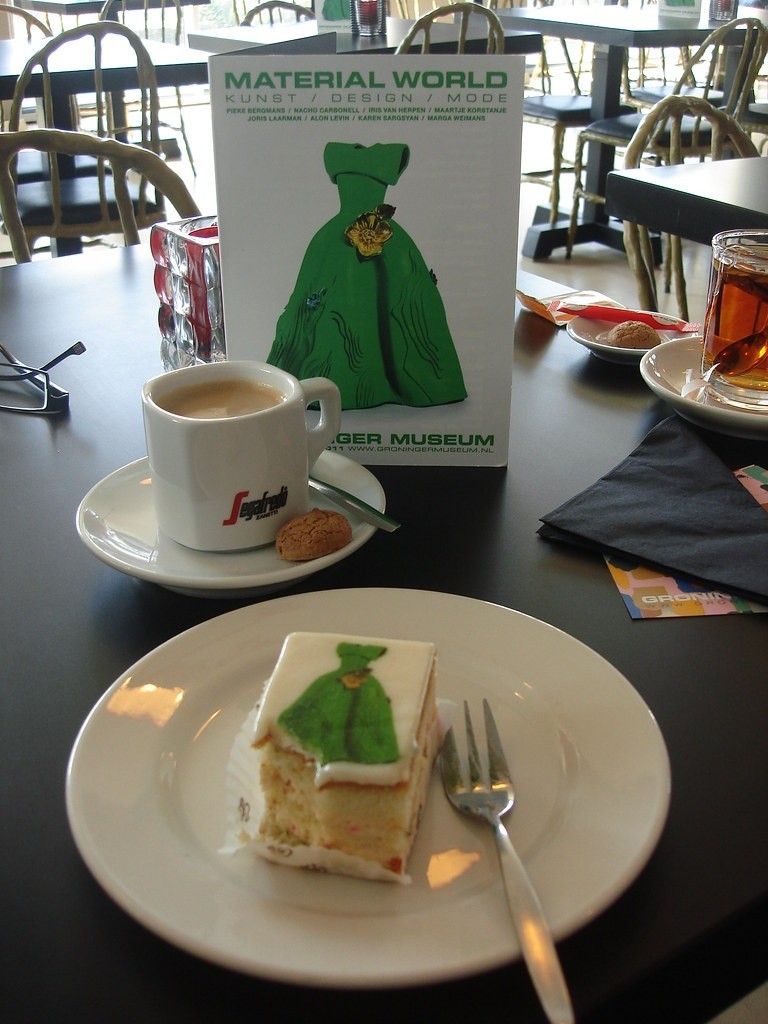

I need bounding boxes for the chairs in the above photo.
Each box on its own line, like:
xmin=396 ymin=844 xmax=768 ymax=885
xmin=0 ymin=0 xmax=768 ymax=327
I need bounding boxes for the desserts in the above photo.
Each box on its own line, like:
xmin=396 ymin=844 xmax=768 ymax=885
xmin=249 ymin=631 xmax=445 ymax=885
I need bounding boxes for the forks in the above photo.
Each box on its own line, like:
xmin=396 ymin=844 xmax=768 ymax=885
xmin=435 ymin=696 xmax=574 ymax=1024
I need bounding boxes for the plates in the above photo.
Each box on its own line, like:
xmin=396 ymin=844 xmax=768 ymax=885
xmin=65 ymin=587 xmax=673 ymax=985
xmin=566 ymin=309 xmax=698 ymax=367
xmin=73 ymin=445 xmax=386 ymax=595
xmin=639 ymin=335 xmax=768 ymax=440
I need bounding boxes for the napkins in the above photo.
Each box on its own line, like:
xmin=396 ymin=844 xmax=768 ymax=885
xmin=538 ymin=414 xmax=768 ymax=581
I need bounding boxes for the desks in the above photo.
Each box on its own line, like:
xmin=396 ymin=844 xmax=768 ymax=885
xmin=0 ymin=213 xmax=768 ymax=1024
xmin=184 ymin=17 xmax=542 ymax=70
xmin=13 ymin=0 xmax=211 ymax=36
xmin=606 ymin=157 xmax=768 ymax=246
xmin=468 ymin=4 xmax=768 ymax=261
xmin=0 ymin=31 xmax=219 ymax=224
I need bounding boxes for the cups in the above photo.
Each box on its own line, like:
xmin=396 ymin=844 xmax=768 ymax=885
xmin=698 ymin=227 xmax=768 ymax=409
xmin=708 ymin=0 xmax=739 ymax=21
xmin=350 ymin=0 xmax=386 ymax=36
xmin=150 ymin=213 xmax=226 ymax=371
xmin=140 ymin=359 xmax=343 ymax=552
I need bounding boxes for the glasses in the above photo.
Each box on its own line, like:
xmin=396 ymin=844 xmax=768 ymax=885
xmin=0 ymin=341 xmax=86 ymax=414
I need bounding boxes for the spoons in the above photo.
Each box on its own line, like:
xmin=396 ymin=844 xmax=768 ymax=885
xmin=712 ymin=315 xmax=768 ymax=375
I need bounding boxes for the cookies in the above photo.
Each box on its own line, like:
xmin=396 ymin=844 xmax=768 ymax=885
xmin=607 ymin=320 xmax=662 ymax=350
xmin=274 ymin=507 xmax=352 ymax=559
